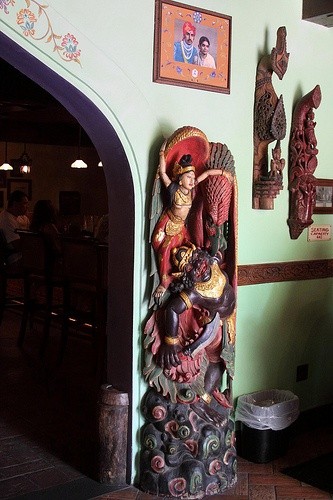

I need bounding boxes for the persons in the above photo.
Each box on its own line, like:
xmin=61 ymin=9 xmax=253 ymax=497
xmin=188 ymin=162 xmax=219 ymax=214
xmin=194 ymin=36 xmax=215 ymax=69
xmin=0 ymin=190 xmax=31 ymax=274
xmin=151 ymin=139 xmax=233 ymax=298
xmin=157 ymin=241 xmax=238 ymax=427
xmin=174 ymin=21 xmax=197 ymax=64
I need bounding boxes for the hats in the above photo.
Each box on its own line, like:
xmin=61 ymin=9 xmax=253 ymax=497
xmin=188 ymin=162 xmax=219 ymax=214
xmin=183 ymin=22 xmax=196 ymax=36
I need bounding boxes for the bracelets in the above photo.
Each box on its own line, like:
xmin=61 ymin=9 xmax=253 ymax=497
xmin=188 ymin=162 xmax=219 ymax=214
xmin=165 ymin=336 xmax=179 ymax=345
xmin=159 ymin=151 xmax=164 ymax=156
xmin=220 ymin=169 xmax=224 ymax=176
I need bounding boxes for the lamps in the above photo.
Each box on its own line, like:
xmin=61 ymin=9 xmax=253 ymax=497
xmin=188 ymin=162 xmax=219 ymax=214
xmin=71 ymin=127 xmax=87 ymax=169
xmin=19 ymin=134 xmax=31 ymax=174
xmin=0 ymin=129 xmax=13 ymax=171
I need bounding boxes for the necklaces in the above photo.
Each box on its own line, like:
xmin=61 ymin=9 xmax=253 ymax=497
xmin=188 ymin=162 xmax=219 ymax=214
xmin=181 ymin=40 xmax=193 ymax=59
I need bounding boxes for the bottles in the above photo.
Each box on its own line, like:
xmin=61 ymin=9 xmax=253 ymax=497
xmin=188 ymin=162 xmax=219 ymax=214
xmin=98 ymin=390 xmax=129 ymax=484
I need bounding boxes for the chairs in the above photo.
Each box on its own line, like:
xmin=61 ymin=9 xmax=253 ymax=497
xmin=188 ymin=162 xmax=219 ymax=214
xmin=14 ymin=227 xmax=61 ymax=344
xmin=0 ymin=228 xmax=24 ymax=322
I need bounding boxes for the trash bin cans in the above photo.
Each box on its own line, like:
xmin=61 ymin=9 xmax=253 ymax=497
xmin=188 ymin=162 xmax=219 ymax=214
xmin=238 ymin=389 xmax=299 ymax=463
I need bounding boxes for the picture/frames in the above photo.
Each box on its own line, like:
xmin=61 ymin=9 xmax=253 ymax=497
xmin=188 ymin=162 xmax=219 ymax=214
xmin=153 ymin=0 xmax=232 ymax=95
